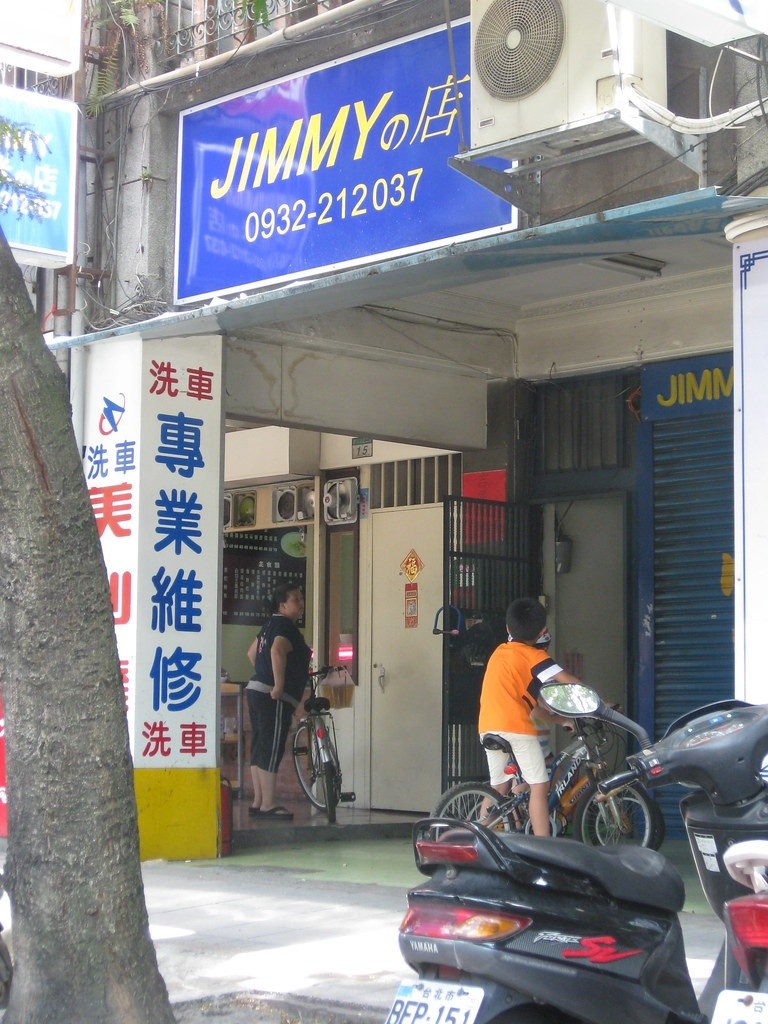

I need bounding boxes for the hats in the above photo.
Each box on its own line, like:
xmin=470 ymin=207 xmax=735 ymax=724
xmin=508 ymin=628 xmax=551 ymax=646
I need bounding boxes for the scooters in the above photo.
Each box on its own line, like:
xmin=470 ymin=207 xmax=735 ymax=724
xmin=386 ymin=697 xmax=768 ymax=1024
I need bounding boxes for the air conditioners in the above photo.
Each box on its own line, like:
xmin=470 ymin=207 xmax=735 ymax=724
xmin=470 ymin=0 xmax=670 ymax=160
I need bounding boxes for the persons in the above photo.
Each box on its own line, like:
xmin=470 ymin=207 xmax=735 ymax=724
xmin=245 ymin=583 xmax=312 ymax=820
xmin=478 ymin=597 xmax=622 ymax=837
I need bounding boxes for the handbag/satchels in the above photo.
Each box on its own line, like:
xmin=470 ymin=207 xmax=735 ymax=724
xmin=316 ymin=665 xmax=355 ymax=710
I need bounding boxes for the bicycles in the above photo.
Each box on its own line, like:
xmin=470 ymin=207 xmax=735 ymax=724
xmin=292 ymin=665 xmax=355 ymax=821
xmin=429 ymin=697 xmax=665 ymax=860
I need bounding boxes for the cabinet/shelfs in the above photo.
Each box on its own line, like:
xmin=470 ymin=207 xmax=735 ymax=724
xmin=218 ymin=684 xmax=243 ymax=800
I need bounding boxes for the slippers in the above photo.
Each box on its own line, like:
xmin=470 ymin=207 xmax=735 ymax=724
xmin=249 ymin=806 xmax=260 ymax=817
xmin=257 ymin=805 xmax=294 ymax=819
